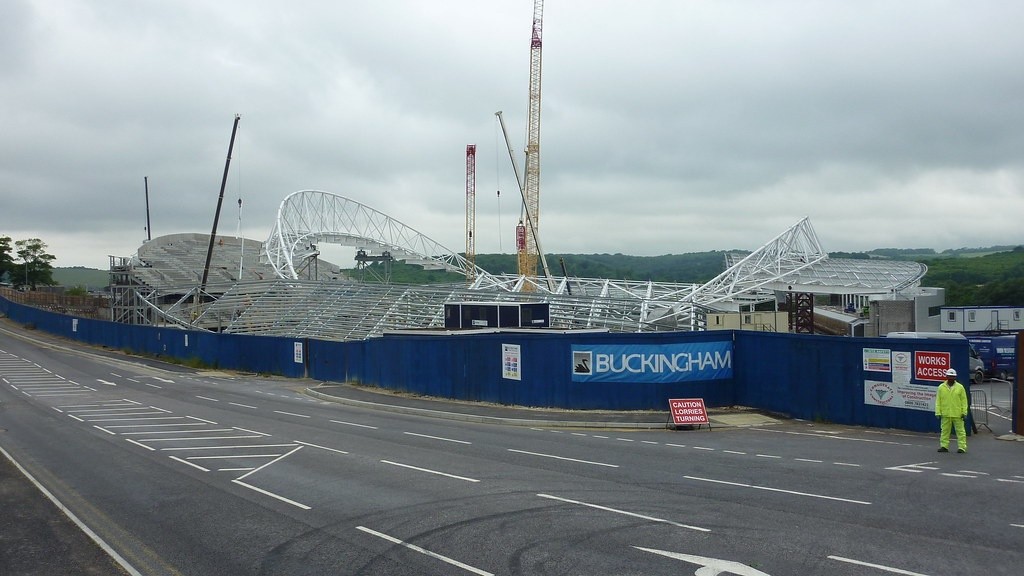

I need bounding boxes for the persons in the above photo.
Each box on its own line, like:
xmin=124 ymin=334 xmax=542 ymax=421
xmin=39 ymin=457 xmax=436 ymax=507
xmin=934 ymin=369 xmax=968 ymax=453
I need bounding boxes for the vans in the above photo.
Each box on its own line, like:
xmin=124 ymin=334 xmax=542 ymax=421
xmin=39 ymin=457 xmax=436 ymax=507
xmin=887 ymin=331 xmax=985 ymax=385
xmin=966 ymin=335 xmax=1016 ymax=380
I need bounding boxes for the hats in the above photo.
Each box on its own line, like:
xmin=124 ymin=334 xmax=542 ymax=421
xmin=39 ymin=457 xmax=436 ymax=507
xmin=945 ymin=368 xmax=957 ymax=376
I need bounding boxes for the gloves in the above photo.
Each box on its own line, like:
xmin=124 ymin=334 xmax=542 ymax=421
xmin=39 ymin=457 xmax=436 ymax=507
xmin=961 ymin=414 xmax=968 ymax=421
xmin=935 ymin=415 xmax=941 ymax=420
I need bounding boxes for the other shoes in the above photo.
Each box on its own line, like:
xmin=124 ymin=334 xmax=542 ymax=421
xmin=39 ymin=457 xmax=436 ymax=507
xmin=937 ymin=447 xmax=949 ymax=453
xmin=957 ymin=449 xmax=964 ymax=453
